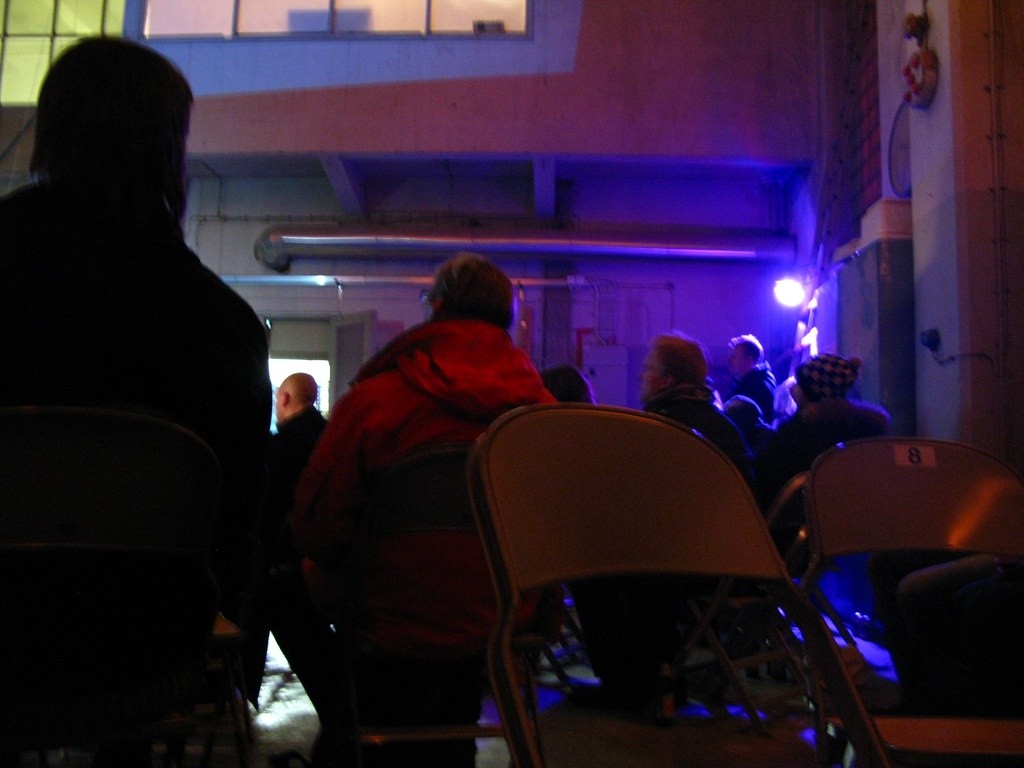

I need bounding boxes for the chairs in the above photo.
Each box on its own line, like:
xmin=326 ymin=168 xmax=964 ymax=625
xmin=0 ymin=409 xmax=258 ymax=768
xmin=337 ymin=443 xmax=551 ymax=768
xmin=464 ymin=403 xmax=892 ymax=768
xmin=798 ymin=435 xmax=1024 ymax=768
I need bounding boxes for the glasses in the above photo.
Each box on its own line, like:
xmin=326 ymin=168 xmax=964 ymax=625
xmin=419 ymin=288 xmax=432 ymax=302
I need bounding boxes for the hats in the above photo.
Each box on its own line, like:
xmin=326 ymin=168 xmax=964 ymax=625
xmin=796 ymin=354 xmax=863 ymax=401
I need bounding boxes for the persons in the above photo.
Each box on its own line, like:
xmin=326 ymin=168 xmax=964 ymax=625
xmin=0 ymin=35 xmax=272 ymax=768
xmin=266 ymin=372 xmax=340 ymax=728
xmin=540 ymin=330 xmax=898 ymax=718
xmin=282 ymin=253 xmax=559 ymax=768
